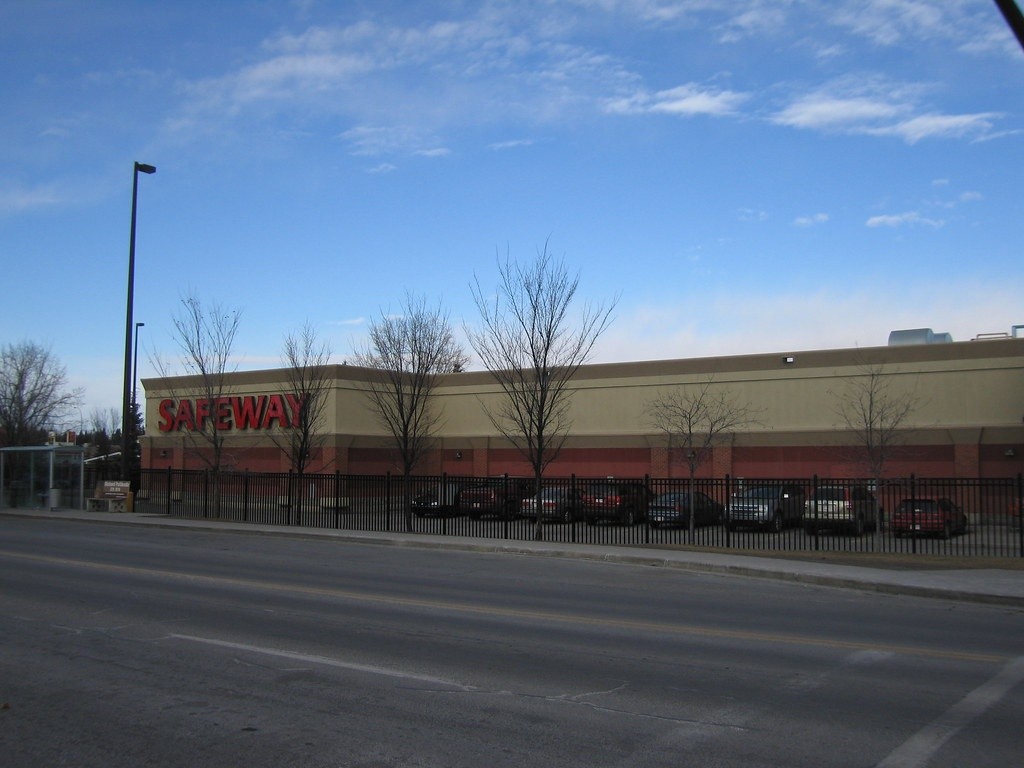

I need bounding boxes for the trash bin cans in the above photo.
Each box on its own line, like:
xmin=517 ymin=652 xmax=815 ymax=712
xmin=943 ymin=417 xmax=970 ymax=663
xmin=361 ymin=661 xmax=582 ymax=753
xmin=48 ymin=488 xmax=63 ymax=512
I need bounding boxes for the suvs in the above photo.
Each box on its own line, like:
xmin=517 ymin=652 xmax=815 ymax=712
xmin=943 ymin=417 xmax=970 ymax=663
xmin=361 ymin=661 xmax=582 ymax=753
xmin=582 ymin=476 xmax=655 ymax=526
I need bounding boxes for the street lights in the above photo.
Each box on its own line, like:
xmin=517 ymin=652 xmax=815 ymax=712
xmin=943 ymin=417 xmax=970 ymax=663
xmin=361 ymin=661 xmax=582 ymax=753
xmin=74 ymin=407 xmax=82 ymax=434
xmin=133 ymin=323 xmax=145 ymax=410
xmin=120 ymin=161 xmax=156 ymax=481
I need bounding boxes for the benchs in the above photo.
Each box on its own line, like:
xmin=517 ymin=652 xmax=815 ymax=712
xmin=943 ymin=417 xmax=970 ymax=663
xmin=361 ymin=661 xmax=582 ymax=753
xmin=87 ymin=480 xmax=130 ymax=513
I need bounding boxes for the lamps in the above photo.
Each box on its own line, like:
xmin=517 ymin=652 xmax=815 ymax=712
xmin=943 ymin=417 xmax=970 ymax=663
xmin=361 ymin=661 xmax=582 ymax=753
xmin=456 ymin=451 xmax=464 ymax=459
xmin=686 ymin=449 xmax=697 ymax=459
xmin=160 ymin=450 xmax=169 ymax=457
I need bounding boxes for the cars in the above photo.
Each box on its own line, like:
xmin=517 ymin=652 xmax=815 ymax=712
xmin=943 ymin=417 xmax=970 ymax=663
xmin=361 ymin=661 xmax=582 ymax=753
xmin=802 ymin=485 xmax=884 ymax=536
xmin=725 ymin=484 xmax=805 ymax=532
xmin=647 ymin=491 xmax=724 ymax=528
xmin=411 ymin=476 xmax=581 ymax=524
xmin=890 ymin=497 xmax=967 ymax=540
